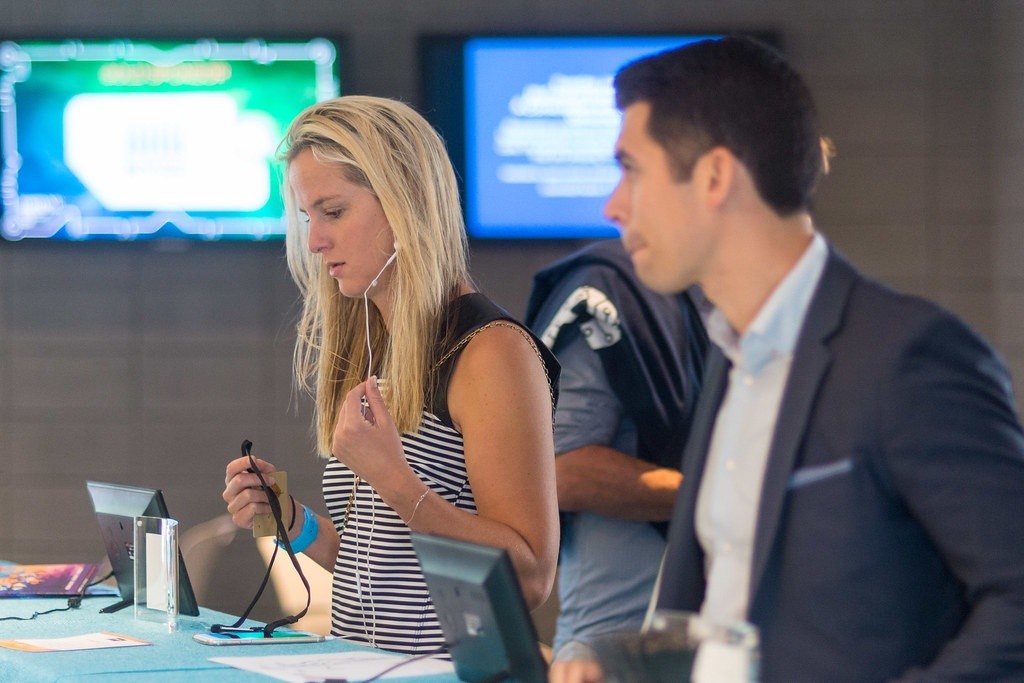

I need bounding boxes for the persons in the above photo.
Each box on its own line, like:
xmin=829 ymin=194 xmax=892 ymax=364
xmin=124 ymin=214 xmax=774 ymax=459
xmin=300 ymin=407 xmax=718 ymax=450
xmin=221 ymin=93 xmax=561 ymax=660
xmin=522 ymin=134 xmax=835 ymax=664
xmin=547 ymin=32 xmax=1024 ymax=683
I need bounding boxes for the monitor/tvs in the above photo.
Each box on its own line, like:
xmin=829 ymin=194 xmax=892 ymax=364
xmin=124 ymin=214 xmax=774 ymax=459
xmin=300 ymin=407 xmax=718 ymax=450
xmin=0 ymin=31 xmax=351 ymax=245
xmin=412 ymin=31 xmax=785 ymax=249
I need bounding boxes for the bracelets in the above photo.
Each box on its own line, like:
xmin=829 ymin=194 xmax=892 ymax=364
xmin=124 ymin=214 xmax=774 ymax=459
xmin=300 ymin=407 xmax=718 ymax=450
xmin=275 ymin=493 xmax=296 ymax=537
xmin=273 ymin=502 xmax=320 ymax=555
xmin=404 ymin=486 xmax=431 ymax=525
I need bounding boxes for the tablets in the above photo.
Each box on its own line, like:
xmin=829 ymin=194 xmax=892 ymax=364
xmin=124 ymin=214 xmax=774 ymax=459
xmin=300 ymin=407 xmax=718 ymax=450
xmin=410 ymin=532 xmax=550 ymax=683
xmin=87 ymin=480 xmax=200 ymax=617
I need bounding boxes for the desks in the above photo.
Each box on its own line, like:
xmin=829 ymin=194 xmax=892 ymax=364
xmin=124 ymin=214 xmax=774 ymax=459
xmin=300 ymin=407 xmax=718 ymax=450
xmin=1 ymin=561 xmax=462 ymax=683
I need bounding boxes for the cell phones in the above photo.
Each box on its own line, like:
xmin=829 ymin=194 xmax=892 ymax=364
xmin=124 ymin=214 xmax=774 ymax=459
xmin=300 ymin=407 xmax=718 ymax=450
xmin=192 ymin=630 xmax=326 ymax=647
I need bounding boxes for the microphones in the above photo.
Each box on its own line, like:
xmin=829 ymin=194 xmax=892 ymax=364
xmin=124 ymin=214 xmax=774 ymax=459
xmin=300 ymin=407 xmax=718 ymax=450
xmin=385 ymin=241 xmax=400 ymax=266
